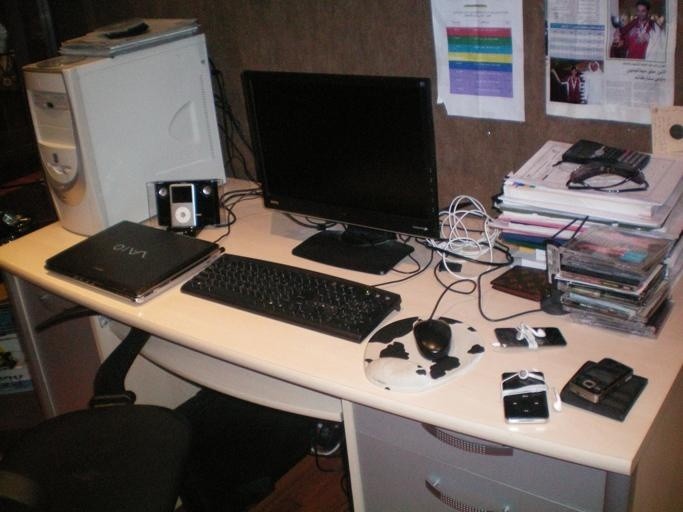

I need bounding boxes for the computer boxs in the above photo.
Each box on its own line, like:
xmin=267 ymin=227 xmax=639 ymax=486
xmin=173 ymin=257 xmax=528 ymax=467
xmin=21 ymin=30 xmax=226 ymax=235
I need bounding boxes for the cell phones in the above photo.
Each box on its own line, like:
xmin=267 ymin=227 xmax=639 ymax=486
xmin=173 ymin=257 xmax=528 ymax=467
xmin=494 ymin=327 xmax=566 ymax=350
xmin=568 ymin=357 xmax=635 ymax=405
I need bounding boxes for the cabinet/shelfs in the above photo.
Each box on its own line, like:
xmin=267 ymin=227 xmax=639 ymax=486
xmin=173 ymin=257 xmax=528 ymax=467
xmin=340 ymin=399 xmax=606 ymax=512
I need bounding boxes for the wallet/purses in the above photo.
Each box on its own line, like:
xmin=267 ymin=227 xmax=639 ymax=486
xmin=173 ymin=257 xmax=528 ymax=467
xmin=491 ymin=265 xmax=554 ymax=301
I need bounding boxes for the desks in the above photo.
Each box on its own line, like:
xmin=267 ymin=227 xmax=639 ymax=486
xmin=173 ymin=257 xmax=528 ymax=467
xmin=1 ymin=178 xmax=682 ymax=511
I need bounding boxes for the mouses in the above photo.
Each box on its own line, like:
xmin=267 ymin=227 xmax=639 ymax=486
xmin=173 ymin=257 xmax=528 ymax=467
xmin=414 ymin=318 xmax=452 ymax=361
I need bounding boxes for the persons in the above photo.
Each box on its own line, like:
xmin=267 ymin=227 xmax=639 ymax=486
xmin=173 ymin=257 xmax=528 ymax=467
xmin=583 ymin=62 xmax=604 ymax=106
xmin=618 ymin=3 xmax=666 ymax=61
xmin=565 ymin=66 xmax=582 ymax=104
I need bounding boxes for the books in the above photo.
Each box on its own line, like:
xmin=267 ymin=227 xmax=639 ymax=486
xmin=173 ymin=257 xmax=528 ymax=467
xmin=488 ymin=137 xmax=681 ymax=301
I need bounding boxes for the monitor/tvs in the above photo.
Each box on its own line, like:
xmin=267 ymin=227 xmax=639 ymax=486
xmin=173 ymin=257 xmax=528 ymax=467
xmin=241 ymin=69 xmax=442 ymax=276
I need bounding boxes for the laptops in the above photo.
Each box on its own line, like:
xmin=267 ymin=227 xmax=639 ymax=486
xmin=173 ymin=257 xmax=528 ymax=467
xmin=44 ymin=219 xmax=219 ymax=304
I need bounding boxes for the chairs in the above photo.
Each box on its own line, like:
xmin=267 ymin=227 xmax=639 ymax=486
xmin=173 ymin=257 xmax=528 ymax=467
xmin=1 ymin=307 xmax=189 ymax=509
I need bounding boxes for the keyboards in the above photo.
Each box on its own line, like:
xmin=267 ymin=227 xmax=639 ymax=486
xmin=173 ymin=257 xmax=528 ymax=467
xmin=181 ymin=253 xmax=402 ymax=344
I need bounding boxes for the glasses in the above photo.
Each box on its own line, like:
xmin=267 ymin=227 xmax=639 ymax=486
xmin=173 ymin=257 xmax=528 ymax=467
xmin=567 ymin=162 xmax=649 ymax=193
